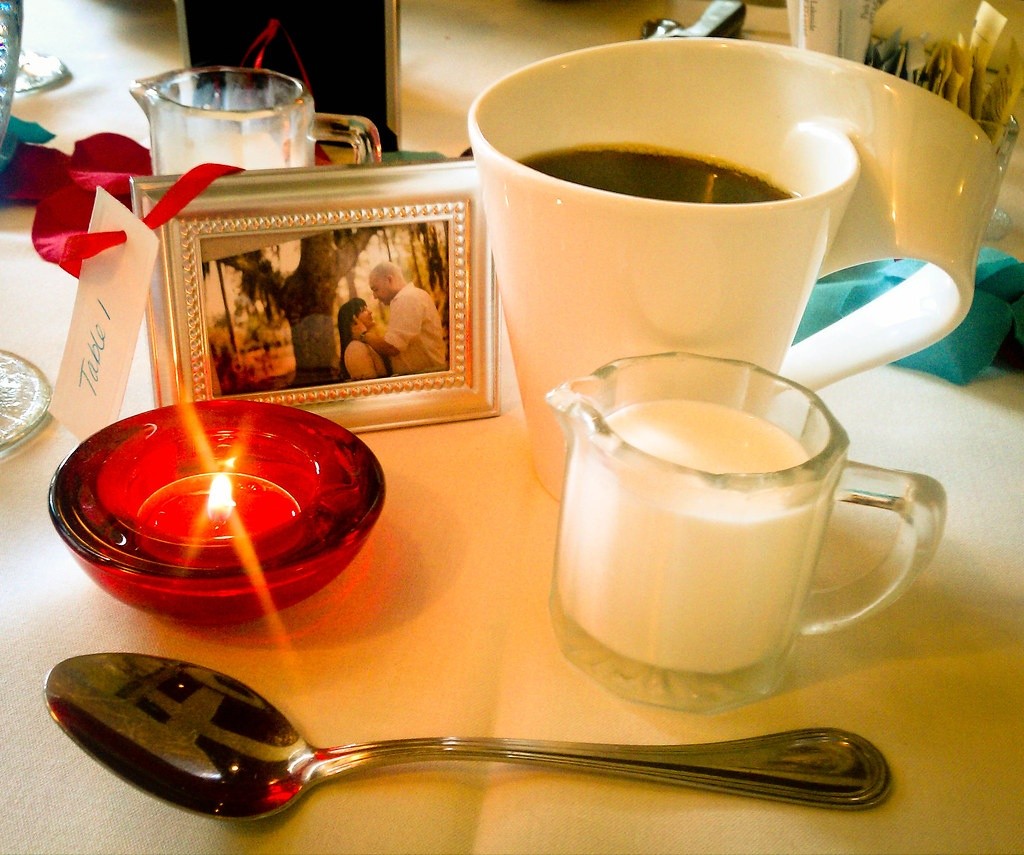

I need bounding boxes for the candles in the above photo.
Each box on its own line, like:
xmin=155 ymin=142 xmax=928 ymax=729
xmin=137 ymin=472 xmax=302 ymax=549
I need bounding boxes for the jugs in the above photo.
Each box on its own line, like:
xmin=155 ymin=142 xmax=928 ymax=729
xmin=465 ymin=37 xmax=1002 ymax=503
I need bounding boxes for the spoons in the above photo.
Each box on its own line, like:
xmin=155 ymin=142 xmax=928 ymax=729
xmin=44 ymin=652 xmax=891 ymax=822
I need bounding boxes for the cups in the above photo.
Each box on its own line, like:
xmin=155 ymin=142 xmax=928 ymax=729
xmin=544 ymin=352 xmax=946 ymax=716
xmin=127 ymin=66 xmax=382 ymax=177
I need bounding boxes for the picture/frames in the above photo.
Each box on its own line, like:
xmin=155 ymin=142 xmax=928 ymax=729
xmin=125 ymin=156 xmax=506 ymax=434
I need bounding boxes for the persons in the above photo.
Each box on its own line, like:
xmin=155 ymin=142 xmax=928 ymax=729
xmin=338 ymin=260 xmax=447 ymax=381
